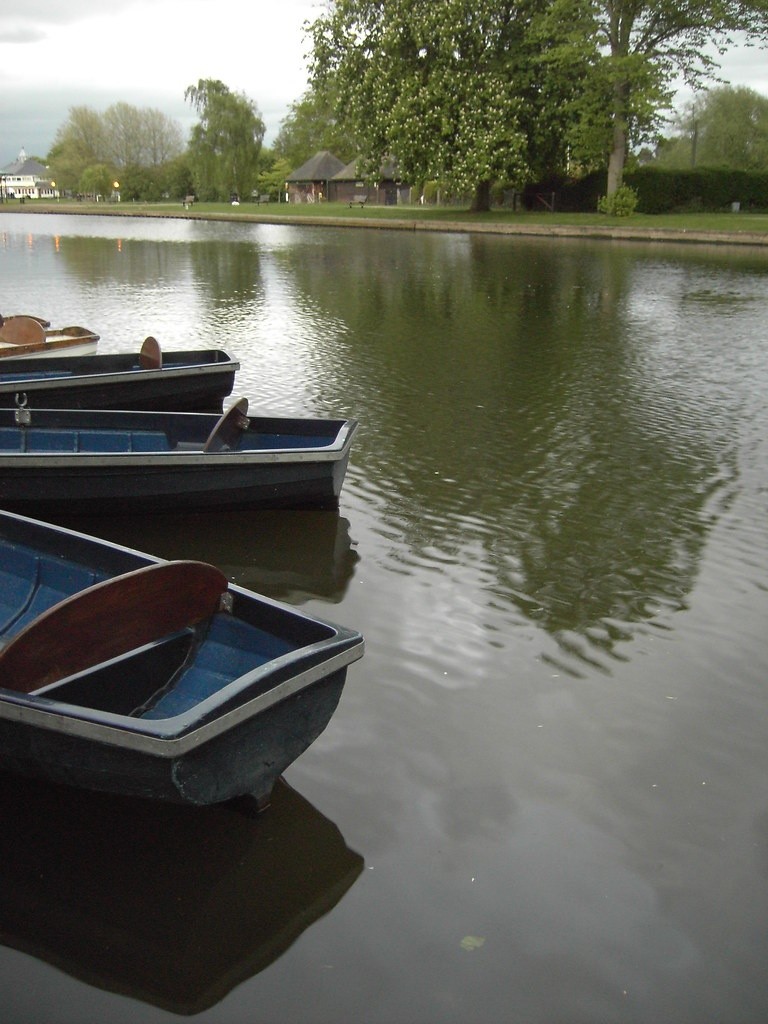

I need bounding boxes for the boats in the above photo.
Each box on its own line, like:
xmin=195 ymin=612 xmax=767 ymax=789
xmin=0 ymin=508 xmax=366 ymax=816
xmin=0 ymin=393 xmax=360 ymax=517
xmin=0 ymin=310 xmax=100 ymax=359
xmin=0 ymin=335 xmax=241 ymax=411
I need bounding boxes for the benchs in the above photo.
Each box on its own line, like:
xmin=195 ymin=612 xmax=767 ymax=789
xmin=256 ymin=195 xmax=270 ymax=206
xmin=182 ymin=195 xmax=195 ymax=208
xmin=348 ymin=194 xmax=368 ymax=209
